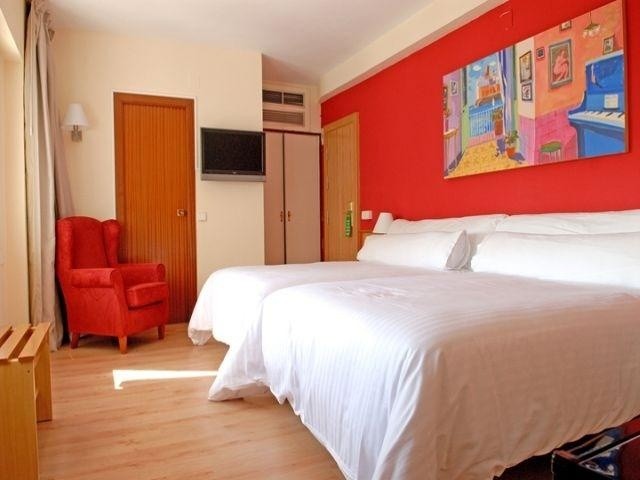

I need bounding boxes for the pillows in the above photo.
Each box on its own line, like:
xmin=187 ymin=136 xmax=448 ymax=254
xmin=356 ymin=230 xmax=469 ymax=271
xmin=470 ymin=230 xmax=640 ymax=288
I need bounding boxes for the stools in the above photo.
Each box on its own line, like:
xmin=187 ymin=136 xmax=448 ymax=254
xmin=0 ymin=322 xmax=54 ymax=480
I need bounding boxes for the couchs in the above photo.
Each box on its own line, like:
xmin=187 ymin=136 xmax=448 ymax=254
xmin=56 ymin=215 xmax=169 ymax=356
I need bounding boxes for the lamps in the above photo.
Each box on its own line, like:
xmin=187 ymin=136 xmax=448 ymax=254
xmin=60 ymin=103 xmax=91 ymax=142
xmin=372 ymin=212 xmax=392 ymax=233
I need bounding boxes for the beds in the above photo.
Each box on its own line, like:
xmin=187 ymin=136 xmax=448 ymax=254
xmin=263 ymin=209 xmax=639 ymax=480
xmin=187 ymin=213 xmax=510 ymax=346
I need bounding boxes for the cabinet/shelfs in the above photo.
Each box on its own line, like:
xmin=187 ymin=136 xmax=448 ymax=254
xmin=263 ymin=128 xmax=321 ymax=264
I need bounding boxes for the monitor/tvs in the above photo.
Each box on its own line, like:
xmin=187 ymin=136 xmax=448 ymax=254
xmin=199 ymin=127 xmax=266 ymax=182
